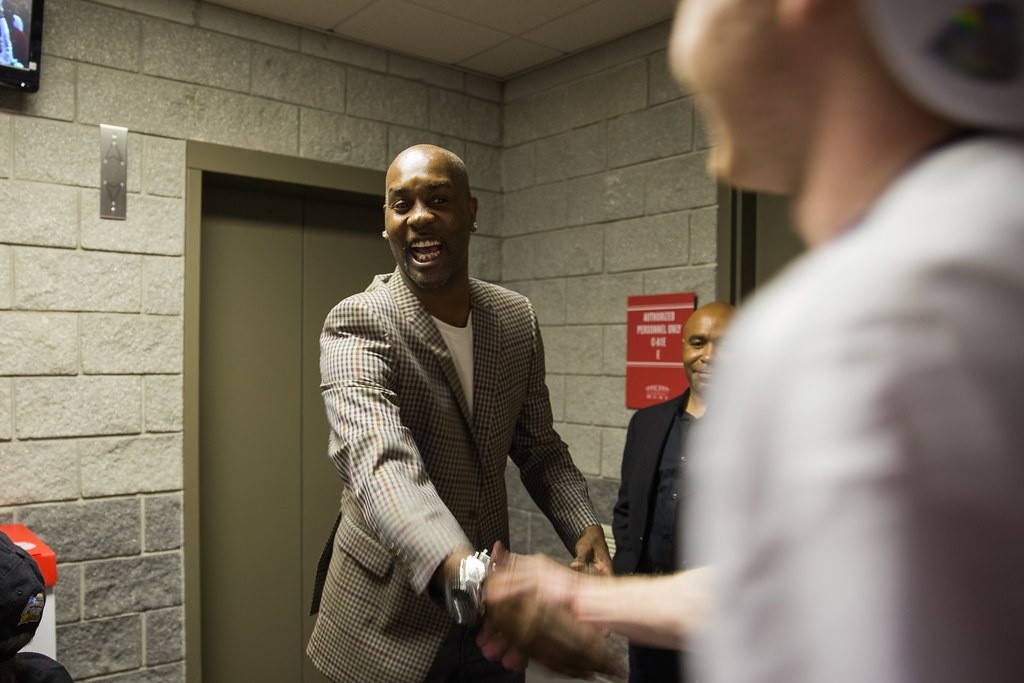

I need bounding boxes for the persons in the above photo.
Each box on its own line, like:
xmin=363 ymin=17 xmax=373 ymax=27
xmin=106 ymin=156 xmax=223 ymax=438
xmin=496 ymin=0 xmax=1023 ymax=683
xmin=611 ymin=302 xmax=754 ymax=680
xmin=0 ymin=531 xmax=76 ymax=683
xmin=303 ymin=141 xmax=618 ymax=682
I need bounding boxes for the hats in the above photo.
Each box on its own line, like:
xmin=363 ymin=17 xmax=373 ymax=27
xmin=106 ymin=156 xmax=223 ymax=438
xmin=865 ymin=0 xmax=1024 ymax=129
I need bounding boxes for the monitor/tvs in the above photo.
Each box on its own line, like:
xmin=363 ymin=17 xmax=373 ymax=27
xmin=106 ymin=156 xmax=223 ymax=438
xmin=0 ymin=0 xmax=44 ymax=93
